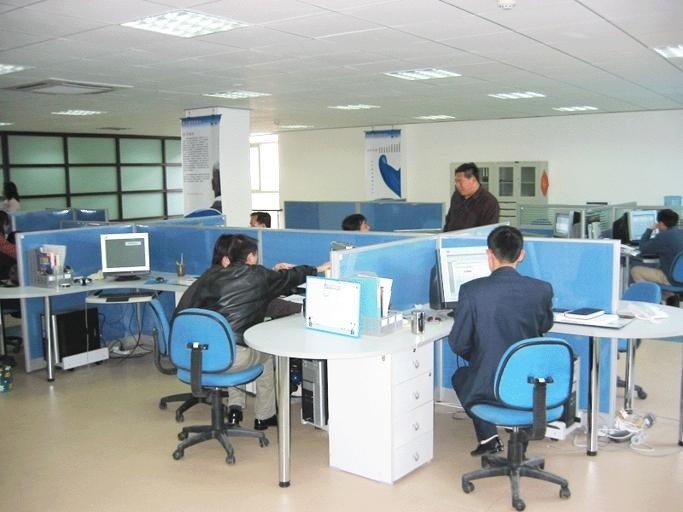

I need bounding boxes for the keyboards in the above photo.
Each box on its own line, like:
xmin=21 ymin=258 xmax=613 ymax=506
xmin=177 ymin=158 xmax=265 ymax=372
xmin=98 ymin=292 xmax=152 ymax=298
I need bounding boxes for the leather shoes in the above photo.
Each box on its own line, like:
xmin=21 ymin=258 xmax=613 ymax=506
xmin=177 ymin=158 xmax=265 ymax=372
xmin=254 ymin=414 xmax=278 ymax=431
xmin=227 ymin=409 xmax=243 ymax=425
xmin=471 ymin=437 xmax=504 ymax=456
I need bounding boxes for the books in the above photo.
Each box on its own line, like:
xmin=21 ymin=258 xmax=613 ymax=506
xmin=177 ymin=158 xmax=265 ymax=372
xmin=37 ymin=253 xmax=59 ymax=276
xmin=565 ymin=307 xmax=605 ymax=321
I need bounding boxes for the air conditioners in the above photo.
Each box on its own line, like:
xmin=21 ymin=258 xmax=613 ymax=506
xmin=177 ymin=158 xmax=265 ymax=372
xmin=0 ymin=76 xmax=132 ymax=96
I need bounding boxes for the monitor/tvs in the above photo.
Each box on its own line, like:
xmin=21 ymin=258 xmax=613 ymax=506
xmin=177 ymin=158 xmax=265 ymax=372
xmin=100 ymin=232 xmax=151 ymax=281
xmin=435 ymin=245 xmax=492 ymax=317
xmin=613 ymin=209 xmax=659 ymax=243
xmin=393 ymin=228 xmax=442 ymax=234
xmin=329 ymin=240 xmax=354 ymax=251
xmin=568 ymin=209 xmax=600 ymax=234
xmin=553 ymin=212 xmax=570 ymax=238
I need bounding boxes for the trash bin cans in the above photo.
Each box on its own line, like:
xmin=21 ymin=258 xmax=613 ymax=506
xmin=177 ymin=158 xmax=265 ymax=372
xmin=0 ymin=355 xmax=17 ymax=394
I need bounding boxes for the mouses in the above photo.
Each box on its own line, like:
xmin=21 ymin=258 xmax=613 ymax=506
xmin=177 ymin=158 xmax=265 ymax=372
xmin=156 ymin=276 xmax=164 ymax=283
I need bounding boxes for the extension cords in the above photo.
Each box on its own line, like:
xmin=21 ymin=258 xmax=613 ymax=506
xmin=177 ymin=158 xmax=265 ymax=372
xmin=644 ymin=413 xmax=655 ymax=427
xmin=112 ymin=345 xmax=130 ymax=356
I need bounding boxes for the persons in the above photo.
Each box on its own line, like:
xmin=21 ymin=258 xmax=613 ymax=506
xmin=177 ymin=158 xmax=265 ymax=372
xmin=0 ymin=181 xmax=21 ymax=210
xmin=249 ymin=212 xmax=272 ymax=228
xmin=628 ymin=209 xmax=683 ymax=308
xmin=192 ymin=234 xmax=331 ymax=429
xmin=342 ymin=214 xmax=370 ymax=231
xmin=448 ymin=225 xmax=554 ymax=457
xmin=443 ymin=162 xmax=500 ymax=232
xmin=0 ymin=211 xmax=17 ymax=292
xmin=168 ymin=234 xmax=230 ymax=313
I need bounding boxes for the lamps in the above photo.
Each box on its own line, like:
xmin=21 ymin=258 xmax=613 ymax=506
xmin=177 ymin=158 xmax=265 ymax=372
xmin=499 ymin=0 xmax=516 ymax=9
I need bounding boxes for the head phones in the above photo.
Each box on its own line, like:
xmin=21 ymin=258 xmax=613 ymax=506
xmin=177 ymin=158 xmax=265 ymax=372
xmin=74 ymin=278 xmax=93 ymax=285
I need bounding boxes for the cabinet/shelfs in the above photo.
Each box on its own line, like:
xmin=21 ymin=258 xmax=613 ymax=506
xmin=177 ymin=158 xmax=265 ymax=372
xmin=449 ymin=160 xmax=550 ymax=228
xmin=327 ymin=345 xmax=434 ymax=486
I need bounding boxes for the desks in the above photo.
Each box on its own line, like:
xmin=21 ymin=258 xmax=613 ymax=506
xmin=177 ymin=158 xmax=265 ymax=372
xmin=1 ymin=272 xmax=683 ymax=489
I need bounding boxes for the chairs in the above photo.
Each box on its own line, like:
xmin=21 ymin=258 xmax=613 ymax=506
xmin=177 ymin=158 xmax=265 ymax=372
xmin=655 ymin=250 xmax=683 ymax=307
xmin=170 ymin=308 xmax=268 ymax=465
xmin=617 ymin=282 xmax=660 ymax=399
xmin=148 ymin=296 xmax=228 ymax=423
xmin=459 ymin=336 xmax=576 ymax=512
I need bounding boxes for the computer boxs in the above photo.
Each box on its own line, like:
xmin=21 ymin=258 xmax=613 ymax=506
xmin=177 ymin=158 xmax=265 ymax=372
xmin=558 ymin=354 xmax=582 ymax=428
xmin=39 ymin=305 xmax=102 ymax=363
xmin=300 ymin=359 xmax=327 ymax=428
